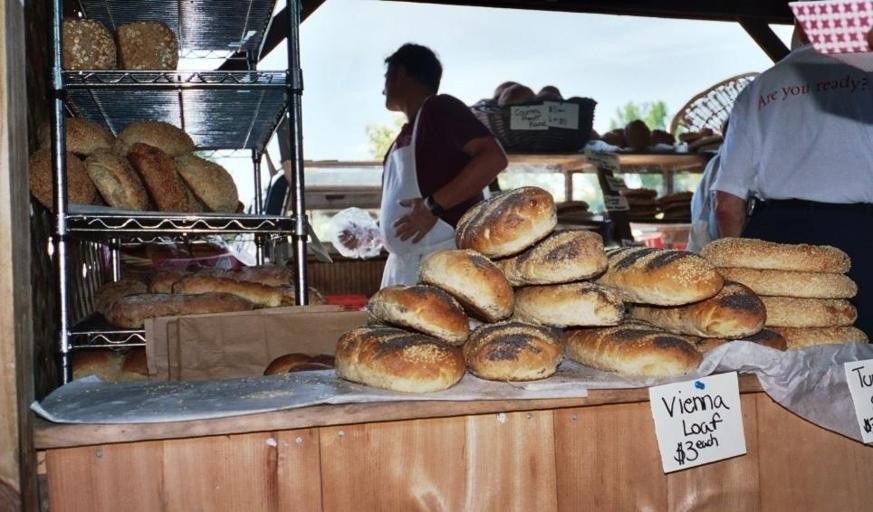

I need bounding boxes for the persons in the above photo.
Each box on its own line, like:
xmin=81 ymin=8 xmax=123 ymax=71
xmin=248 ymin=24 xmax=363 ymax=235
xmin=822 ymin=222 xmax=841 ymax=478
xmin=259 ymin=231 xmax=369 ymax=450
xmin=686 ymin=114 xmax=753 ymax=259
xmin=708 ymin=15 xmax=873 ymax=343
xmin=380 ymin=43 xmax=508 ymax=291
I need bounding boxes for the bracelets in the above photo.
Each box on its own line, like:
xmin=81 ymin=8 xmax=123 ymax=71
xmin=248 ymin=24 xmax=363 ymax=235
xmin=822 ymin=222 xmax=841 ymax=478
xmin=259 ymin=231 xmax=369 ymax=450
xmin=424 ymin=194 xmax=445 ymax=218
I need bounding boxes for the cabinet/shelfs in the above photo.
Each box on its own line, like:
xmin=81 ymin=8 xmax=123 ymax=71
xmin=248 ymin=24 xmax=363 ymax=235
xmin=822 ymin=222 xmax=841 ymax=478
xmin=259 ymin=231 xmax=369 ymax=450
xmin=51 ymin=0 xmax=307 ymax=385
xmin=506 ymin=153 xmax=717 ymax=255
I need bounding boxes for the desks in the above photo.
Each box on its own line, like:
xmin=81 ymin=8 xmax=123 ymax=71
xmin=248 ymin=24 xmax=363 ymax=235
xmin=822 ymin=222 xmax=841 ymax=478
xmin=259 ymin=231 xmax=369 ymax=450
xmin=31 ymin=373 xmax=872 ymax=512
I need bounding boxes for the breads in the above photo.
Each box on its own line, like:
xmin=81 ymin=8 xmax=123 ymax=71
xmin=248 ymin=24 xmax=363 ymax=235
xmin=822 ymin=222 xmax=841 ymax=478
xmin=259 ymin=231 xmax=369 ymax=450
xmin=58 ymin=16 xmax=178 ymax=74
xmin=680 ymin=127 xmax=723 ymax=151
xmin=71 ymin=347 xmax=149 ymax=382
xmin=29 ymin=116 xmax=244 ymax=213
xmin=334 ymin=186 xmax=872 ymax=393
xmin=262 ymin=349 xmax=334 ymax=374
xmin=91 ymin=243 xmax=327 ymax=329
xmin=592 ymin=120 xmax=676 ymax=149
xmin=492 ymin=81 xmax=563 ymax=106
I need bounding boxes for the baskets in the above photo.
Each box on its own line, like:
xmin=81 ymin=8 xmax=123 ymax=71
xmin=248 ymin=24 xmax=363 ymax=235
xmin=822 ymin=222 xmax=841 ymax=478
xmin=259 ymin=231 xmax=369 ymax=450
xmin=305 ymin=249 xmax=388 ymax=298
xmin=468 ymin=98 xmax=597 ymax=154
